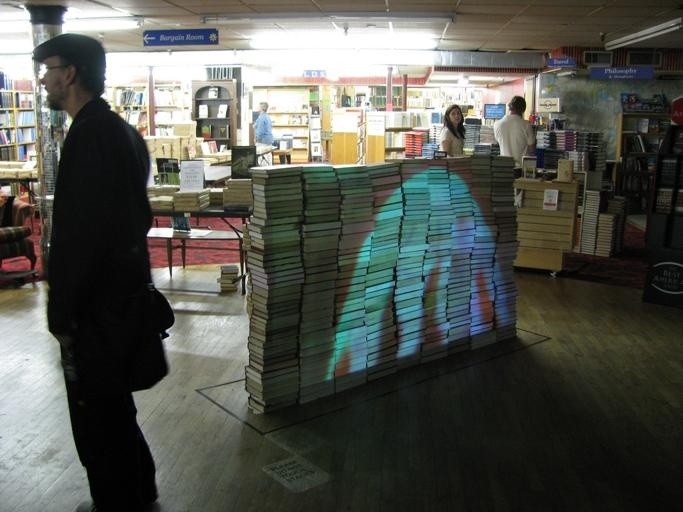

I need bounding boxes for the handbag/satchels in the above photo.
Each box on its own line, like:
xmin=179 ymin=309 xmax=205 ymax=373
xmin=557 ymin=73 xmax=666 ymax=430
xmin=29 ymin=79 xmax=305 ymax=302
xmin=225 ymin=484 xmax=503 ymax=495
xmin=136 ymin=285 xmax=175 ymax=390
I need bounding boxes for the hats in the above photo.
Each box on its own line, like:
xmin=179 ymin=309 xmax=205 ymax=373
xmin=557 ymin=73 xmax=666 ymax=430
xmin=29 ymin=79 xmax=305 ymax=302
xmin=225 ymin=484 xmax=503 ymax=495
xmin=32 ymin=33 xmax=106 ymax=81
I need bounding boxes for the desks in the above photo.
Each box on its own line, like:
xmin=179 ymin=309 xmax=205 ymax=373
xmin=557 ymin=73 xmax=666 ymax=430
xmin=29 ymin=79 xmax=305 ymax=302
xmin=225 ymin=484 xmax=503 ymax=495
xmin=148 ymin=204 xmax=254 ymax=295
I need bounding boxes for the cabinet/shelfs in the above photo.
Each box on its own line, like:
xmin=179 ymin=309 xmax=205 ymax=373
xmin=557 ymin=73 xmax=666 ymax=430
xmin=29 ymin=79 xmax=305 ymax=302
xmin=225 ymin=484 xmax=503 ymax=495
xmin=610 ymin=112 xmax=683 ymax=219
xmin=0 ymin=79 xmax=238 ymax=196
xmin=266 ymin=111 xmax=309 ymax=150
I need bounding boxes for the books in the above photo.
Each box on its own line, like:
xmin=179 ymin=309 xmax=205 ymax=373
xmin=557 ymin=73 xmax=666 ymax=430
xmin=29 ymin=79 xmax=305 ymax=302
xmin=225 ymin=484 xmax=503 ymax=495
xmin=0 ymin=69 xmax=73 ymax=197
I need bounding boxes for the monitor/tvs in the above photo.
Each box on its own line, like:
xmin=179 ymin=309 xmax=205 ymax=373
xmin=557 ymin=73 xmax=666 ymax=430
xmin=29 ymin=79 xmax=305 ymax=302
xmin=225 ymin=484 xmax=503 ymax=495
xmin=484 ymin=102 xmax=506 ymax=120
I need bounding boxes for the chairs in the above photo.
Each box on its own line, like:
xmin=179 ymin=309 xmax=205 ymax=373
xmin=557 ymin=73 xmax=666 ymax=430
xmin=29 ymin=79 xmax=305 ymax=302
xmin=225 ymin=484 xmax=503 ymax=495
xmin=0 ymin=193 xmax=38 ymax=287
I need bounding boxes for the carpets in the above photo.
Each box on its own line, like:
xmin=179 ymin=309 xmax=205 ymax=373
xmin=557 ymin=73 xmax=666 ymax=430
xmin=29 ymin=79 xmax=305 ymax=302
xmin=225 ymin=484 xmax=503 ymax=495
xmin=0 ymin=213 xmax=252 ymax=288
xmin=562 ymin=218 xmax=645 ymax=289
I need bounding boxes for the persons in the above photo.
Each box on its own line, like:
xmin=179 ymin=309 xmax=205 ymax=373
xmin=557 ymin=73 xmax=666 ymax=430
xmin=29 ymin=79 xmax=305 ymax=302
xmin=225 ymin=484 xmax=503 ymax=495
xmin=32 ymin=33 xmax=169 ymax=512
